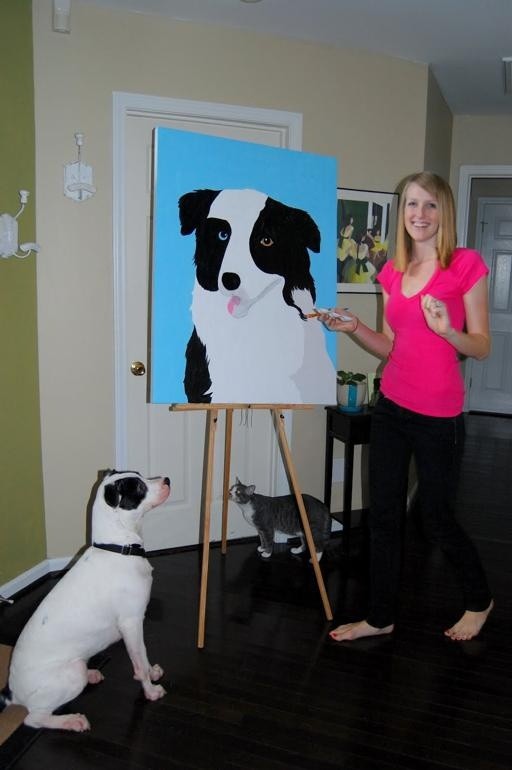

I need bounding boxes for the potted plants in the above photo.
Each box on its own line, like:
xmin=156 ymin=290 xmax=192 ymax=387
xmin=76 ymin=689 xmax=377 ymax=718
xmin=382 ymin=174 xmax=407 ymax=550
xmin=336 ymin=371 xmax=369 ymax=415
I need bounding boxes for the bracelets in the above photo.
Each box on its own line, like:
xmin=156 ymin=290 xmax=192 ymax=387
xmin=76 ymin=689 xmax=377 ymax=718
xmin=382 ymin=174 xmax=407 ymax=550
xmin=351 ymin=317 xmax=359 ymax=335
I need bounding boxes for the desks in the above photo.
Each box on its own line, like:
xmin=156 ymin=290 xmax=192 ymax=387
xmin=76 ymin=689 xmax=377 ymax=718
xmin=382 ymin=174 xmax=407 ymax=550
xmin=324 ymin=402 xmax=377 ymax=559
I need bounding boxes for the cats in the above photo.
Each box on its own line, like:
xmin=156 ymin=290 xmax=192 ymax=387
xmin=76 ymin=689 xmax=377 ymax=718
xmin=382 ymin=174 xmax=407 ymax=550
xmin=229 ymin=476 xmax=333 ymax=564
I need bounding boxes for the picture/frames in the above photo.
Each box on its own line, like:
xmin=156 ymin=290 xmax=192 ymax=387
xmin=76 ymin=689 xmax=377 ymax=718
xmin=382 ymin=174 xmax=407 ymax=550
xmin=335 ymin=186 xmax=402 ymax=296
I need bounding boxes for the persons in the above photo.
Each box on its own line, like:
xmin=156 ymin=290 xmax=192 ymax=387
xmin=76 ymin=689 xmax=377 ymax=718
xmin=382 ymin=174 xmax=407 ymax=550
xmin=317 ymin=170 xmax=491 ymax=642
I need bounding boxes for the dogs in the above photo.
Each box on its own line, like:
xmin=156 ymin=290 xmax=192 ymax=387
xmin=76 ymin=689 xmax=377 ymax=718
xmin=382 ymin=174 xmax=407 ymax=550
xmin=0 ymin=469 xmax=171 ymax=734
xmin=177 ymin=187 xmax=336 ymax=405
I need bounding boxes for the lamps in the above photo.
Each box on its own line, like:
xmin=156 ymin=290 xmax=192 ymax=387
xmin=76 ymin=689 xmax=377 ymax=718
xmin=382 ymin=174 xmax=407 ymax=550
xmin=65 ymin=132 xmax=95 ymax=202
xmin=1 ymin=187 xmax=43 ymax=263
